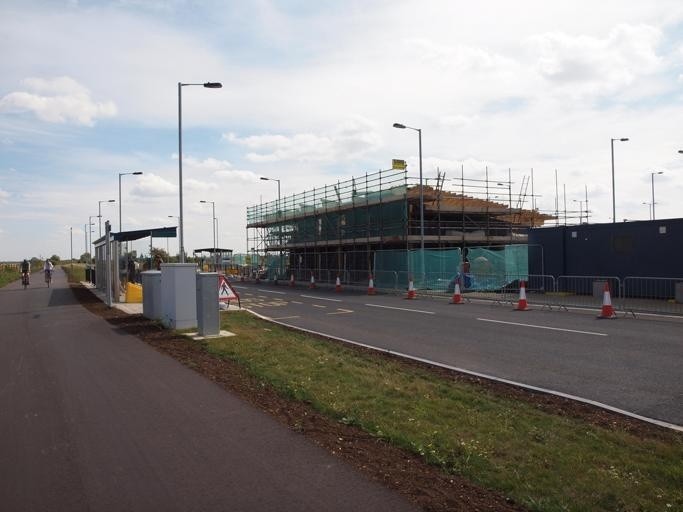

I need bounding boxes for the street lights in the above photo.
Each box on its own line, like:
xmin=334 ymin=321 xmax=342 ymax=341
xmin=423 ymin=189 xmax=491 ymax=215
xmin=611 ymin=138 xmax=629 ymax=222
xmin=573 ymin=200 xmax=588 ymax=224
xmin=85 ymin=171 xmax=143 ymax=275
xmin=178 ymin=81 xmax=223 ymax=268
xmin=260 ymin=177 xmax=280 ymax=211
xmin=199 ymin=201 xmax=219 ymax=269
xmin=642 ymin=172 xmax=663 ymax=220
xmin=168 ymin=216 xmax=181 ymax=264
xmin=393 ymin=123 xmax=426 ymax=289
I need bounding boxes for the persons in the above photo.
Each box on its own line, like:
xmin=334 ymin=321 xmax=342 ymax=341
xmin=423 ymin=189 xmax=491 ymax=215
xmin=20 ymin=260 xmax=30 ymax=285
xmin=127 ymin=255 xmax=163 ymax=283
xmin=44 ymin=260 xmax=53 ymax=284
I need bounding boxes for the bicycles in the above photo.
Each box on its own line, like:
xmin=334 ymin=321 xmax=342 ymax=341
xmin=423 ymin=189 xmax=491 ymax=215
xmin=22 ymin=271 xmax=30 ymax=289
xmin=44 ymin=269 xmax=53 ymax=288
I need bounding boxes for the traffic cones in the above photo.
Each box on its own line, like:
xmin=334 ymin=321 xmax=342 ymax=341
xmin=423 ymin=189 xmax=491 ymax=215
xmin=449 ymin=279 xmax=465 ymax=304
xmin=513 ymin=280 xmax=533 ymax=310
xmin=407 ymin=277 xmax=418 ymax=299
xmin=240 ymin=270 xmax=376 ymax=296
xmin=596 ymin=282 xmax=618 ymax=319
